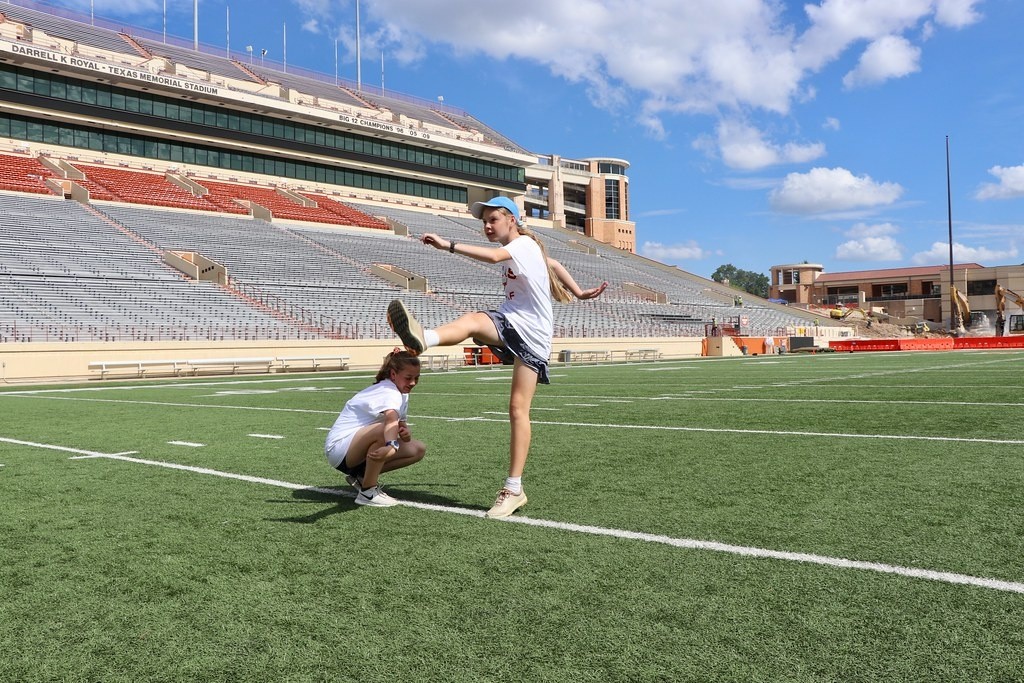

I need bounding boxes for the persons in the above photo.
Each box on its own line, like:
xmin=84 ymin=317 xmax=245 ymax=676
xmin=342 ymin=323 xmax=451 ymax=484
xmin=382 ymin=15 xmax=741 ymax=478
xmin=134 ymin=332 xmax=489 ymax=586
xmin=814 ymin=317 xmax=819 ymax=326
xmin=866 ymin=319 xmax=871 ymax=328
xmin=734 ymin=294 xmax=742 ymax=306
xmin=387 ymin=196 xmax=608 ymax=519
xmin=711 ymin=317 xmax=718 ymax=336
xmin=325 ymin=347 xmax=426 ymax=507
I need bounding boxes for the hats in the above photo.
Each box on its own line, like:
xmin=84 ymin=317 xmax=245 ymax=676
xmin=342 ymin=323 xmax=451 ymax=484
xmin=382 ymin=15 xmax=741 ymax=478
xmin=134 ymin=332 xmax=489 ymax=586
xmin=471 ymin=196 xmax=519 ymax=223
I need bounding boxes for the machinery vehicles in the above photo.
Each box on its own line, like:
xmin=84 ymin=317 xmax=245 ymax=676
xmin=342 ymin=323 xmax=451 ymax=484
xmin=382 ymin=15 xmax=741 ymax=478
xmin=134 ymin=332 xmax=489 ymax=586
xmin=994 ymin=285 xmax=1024 ymax=337
xmin=831 ymin=306 xmax=868 ymax=321
xmin=911 ymin=322 xmax=930 ymax=337
xmin=951 ymin=284 xmax=994 ymax=337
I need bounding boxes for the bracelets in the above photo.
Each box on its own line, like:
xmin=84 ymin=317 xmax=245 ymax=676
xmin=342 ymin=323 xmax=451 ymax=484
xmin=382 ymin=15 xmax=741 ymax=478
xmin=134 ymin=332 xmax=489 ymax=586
xmin=450 ymin=241 xmax=455 ymax=253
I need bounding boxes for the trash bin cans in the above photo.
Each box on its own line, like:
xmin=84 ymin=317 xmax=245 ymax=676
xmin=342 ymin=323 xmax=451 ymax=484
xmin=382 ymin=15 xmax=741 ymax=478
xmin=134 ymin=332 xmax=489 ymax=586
xmin=562 ymin=350 xmax=571 ymax=362
xmin=464 ymin=346 xmax=503 ymax=364
xmin=781 ymin=346 xmax=786 ymax=353
xmin=743 ymin=345 xmax=747 ymax=354
xmin=774 ymin=346 xmax=779 ymax=356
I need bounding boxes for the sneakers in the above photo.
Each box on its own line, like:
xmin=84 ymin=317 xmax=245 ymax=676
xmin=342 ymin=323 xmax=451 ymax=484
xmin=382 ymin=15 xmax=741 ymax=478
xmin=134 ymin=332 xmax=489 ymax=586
xmin=486 ymin=483 xmax=528 ymax=519
xmin=345 ymin=473 xmax=366 ymax=491
xmin=355 ymin=483 xmax=397 ymax=508
xmin=387 ymin=298 xmax=428 ymax=357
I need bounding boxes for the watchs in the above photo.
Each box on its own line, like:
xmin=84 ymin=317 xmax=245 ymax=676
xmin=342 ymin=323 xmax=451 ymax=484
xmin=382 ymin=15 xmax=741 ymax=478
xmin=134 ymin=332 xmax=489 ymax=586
xmin=386 ymin=440 xmax=399 ymax=451
